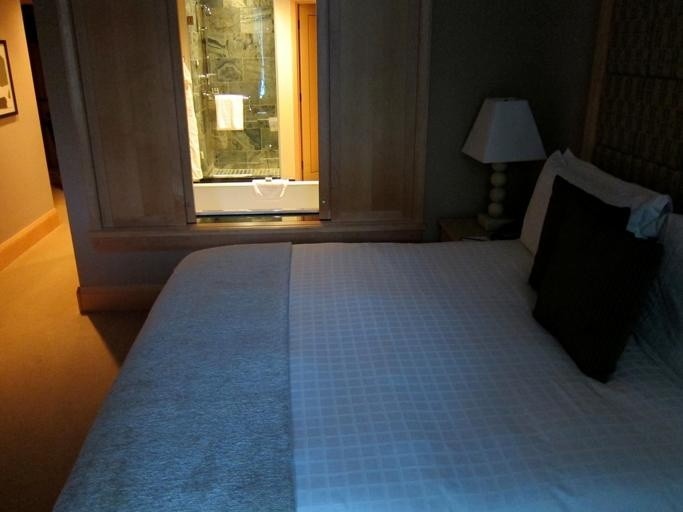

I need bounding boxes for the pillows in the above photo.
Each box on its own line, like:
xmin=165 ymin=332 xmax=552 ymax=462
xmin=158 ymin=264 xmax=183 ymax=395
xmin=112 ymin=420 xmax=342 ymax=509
xmin=520 ymin=147 xmax=683 ymax=387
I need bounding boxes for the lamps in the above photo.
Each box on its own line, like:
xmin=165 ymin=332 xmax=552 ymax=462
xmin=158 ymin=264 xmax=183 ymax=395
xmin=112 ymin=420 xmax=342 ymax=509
xmin=461 ymin=97 xmax=548 ymax=239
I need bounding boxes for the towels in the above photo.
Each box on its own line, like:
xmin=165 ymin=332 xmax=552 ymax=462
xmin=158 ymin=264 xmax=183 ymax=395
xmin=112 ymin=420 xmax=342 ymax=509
xmin=215 ymin=95 xmax=244 ymax=130
xmin=252 ymin=180 xmax=289 ymax=200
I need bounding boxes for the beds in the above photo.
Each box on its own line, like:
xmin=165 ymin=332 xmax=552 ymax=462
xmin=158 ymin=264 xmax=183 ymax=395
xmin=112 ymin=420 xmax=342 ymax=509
xmin=52 ymin=242 xmax=683 ymax=512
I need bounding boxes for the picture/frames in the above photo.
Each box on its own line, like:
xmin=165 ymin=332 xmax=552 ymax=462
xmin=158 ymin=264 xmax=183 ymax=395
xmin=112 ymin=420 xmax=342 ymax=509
xmin=0 ymin=40 xmax=18 ymax=120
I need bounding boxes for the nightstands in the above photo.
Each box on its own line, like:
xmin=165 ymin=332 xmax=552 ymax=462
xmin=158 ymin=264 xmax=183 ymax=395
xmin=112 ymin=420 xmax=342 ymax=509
xmin=438 ymin=218 xmax=495 ymax=242
